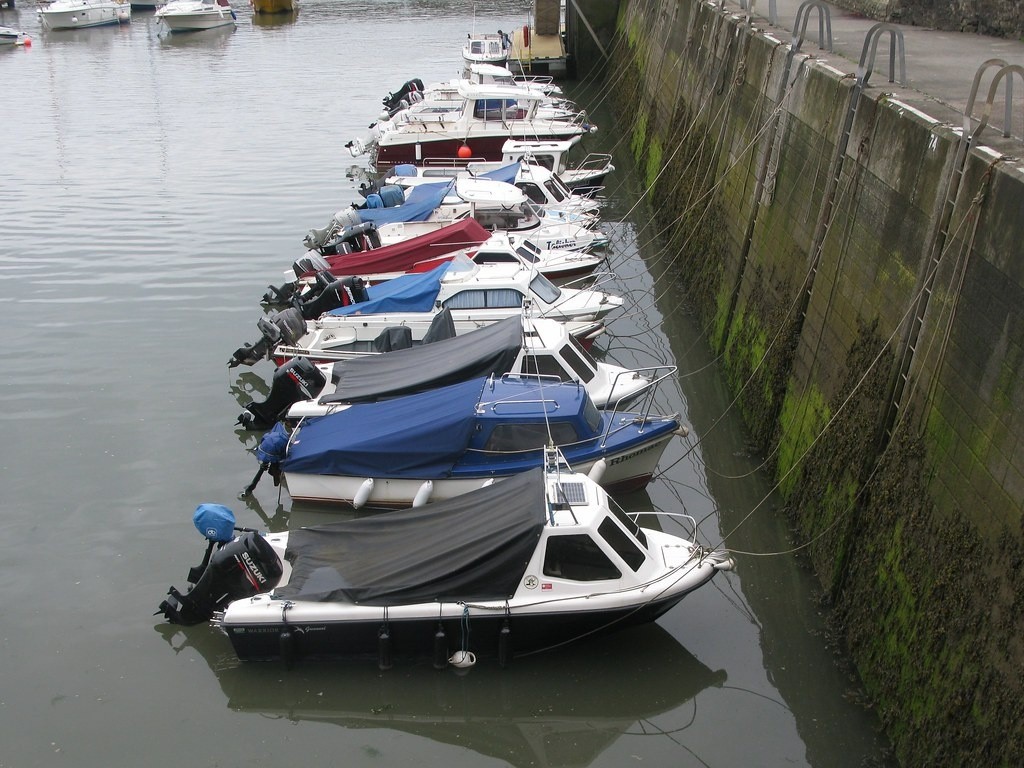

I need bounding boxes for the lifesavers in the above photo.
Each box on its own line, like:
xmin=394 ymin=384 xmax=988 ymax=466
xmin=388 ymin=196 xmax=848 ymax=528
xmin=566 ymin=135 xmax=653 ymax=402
xmin=522 ymin=25 xmax=529 ymax=48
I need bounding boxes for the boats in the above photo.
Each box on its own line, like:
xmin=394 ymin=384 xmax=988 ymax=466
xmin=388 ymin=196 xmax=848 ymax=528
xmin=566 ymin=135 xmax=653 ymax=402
xmin=461 ymin=36 xmax=509 ymax=71
xmin=250 ymin=0 xmax=298 ymax=14
xmin=37 ymin=0 xmax=132 ymax=31
xmin=162 ymin=466 xmax=720 ymax=665
xmin=0 ymin=26 xmax=18 ymax=45
xmin=284 ymin=63 xmax=615 ymax=290
xmin=154 ymin=0 xmax=236 ymax=32
xmin=240 ymin=372 xmax=681 ymax=511
xmin=235 ymin=313 xmax=652 ymax=432
xmin=259 ymin=251 xmax=623 ymax=349
